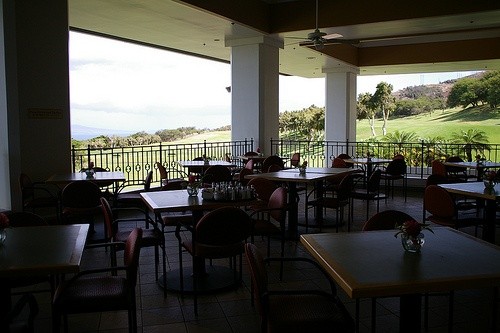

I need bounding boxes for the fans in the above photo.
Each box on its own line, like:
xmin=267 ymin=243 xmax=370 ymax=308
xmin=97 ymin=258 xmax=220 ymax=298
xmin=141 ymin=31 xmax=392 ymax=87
xmin=280 ymin=0 xmax=360 ymax=48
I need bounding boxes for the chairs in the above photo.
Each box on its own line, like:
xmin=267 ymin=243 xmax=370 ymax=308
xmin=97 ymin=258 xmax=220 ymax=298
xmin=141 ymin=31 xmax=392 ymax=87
xmin=0 ymin=152 xmax=500 ymax=333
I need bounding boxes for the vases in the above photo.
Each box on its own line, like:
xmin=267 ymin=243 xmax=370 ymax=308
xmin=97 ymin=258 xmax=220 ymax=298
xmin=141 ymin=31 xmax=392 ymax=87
xmin=401 ymin=233 xmax=425 ymax=253
xmin=187 ymin=185 xmax=199 ymax=196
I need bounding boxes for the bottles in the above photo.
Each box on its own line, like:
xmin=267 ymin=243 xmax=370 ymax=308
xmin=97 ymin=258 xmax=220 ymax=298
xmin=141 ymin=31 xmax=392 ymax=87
xmin=210 ymin=182 xmax=257 ymax=200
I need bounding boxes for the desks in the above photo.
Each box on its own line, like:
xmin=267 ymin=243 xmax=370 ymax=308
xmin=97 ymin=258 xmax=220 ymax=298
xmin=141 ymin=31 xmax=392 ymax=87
xmin=183 ymin=160 xmax=237 ymax=177
xmin=343 ymin=157 xmax=393 ymax=200
xmin=139 ymin=188 xmax=264 ymax=296
xmin=46 ymin=171 xmax=125 ymax=234
xmin=443 ymin=161 xmax=500 ymax=182
xmin=0 ymin=223 xmax=90 ymax=333
xmin=244 ymin=166 xmax=363 ymax=241
xmin=300 ymin=227 xmax=500 ymax=333
xmin=237 ymin=155 xmax=289 ymax=174
xmin=437 ymin=181 xmax=500 ymax=245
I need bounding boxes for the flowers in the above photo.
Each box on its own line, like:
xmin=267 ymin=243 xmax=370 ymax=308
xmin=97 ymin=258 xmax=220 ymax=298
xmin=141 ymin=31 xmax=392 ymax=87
xmin=188 ymin=175 xmax=198 ymax=193
xmin=394 ymin=221 xmax=435 ymax=240
